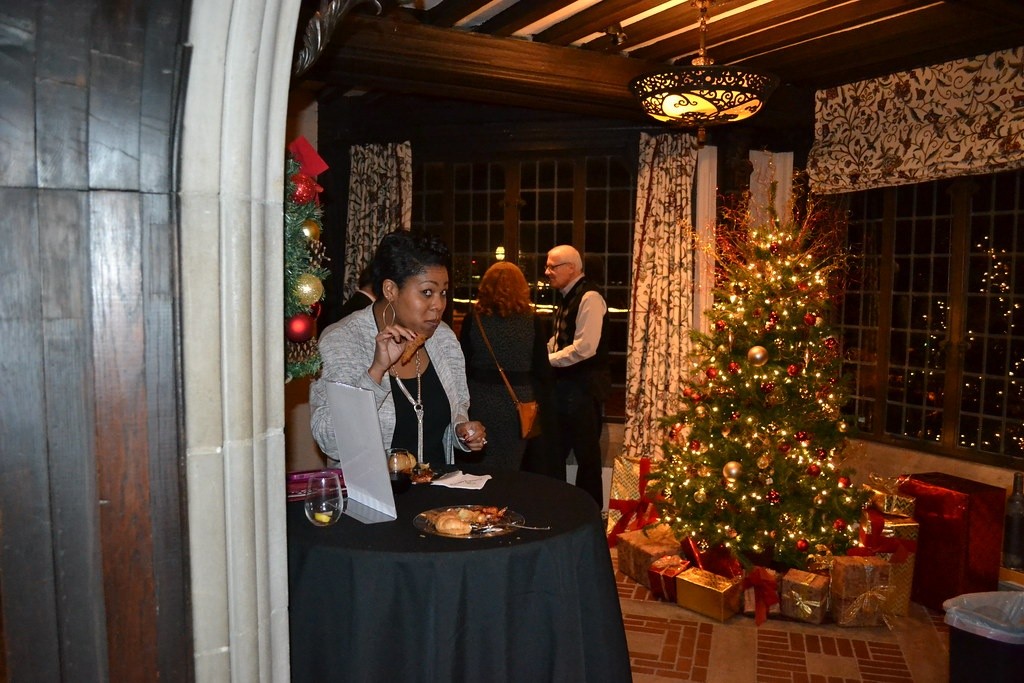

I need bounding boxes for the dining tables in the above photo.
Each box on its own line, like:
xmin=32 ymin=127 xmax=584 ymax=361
xmin=287 ymin=464 xmax=632 ymax=683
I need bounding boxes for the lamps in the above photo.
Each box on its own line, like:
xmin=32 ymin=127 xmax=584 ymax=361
xmin=628 ymin=0 xmax=776 ymax=129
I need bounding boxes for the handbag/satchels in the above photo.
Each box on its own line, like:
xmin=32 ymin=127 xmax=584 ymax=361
xmin=285 ymin=468 xmax=347 ymax=502
xmin=514 ymin=401 xmax=541 ymax=437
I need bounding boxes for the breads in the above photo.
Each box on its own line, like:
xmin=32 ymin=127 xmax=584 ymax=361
xmin=434 ymin=509 xmax=473 ymax=535
xmin=388 ymin=452 xmax=417 ymax=473
xmin=401 ymin=333 xmax=426 ymax=364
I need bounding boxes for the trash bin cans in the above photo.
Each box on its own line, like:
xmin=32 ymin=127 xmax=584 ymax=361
xmin=943 ymin=590 xmax=1024 ymax=683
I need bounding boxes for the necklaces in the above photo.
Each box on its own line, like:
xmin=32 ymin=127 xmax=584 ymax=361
xmin=392 ymin=350 xmax=424 ymax=463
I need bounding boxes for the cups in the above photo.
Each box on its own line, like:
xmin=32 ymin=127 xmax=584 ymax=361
xmin=384 ymin=448 xmax=411 ymax=482
xmin=304 ymin=473 xmax=343 ymax=527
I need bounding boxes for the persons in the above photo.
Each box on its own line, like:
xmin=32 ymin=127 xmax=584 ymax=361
xmin=459 ymin=262 xmax=548 ymax=470
xmin=309 ymin=223 xmax=487 ymax=497
xmin=336 ymin=264 xmax=377 ymax=322
xmin=545 ymin=245 xmax=610 ymax=513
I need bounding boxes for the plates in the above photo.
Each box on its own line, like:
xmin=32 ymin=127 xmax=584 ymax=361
xmin=412 ymin=505 xmax=525 ymax=538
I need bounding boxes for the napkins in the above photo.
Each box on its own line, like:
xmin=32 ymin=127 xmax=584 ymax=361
xmin=430 ymin=470 xmax=492 ymax=490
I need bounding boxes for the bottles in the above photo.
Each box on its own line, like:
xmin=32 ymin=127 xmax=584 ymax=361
xmin=1002 ymin=471 xmax=1024 ymax=572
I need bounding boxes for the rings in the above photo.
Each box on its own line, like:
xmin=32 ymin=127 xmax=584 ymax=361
xmin=482 ymin=438 xmax=488 ymax=444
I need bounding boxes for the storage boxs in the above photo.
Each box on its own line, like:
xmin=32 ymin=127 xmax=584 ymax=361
xmin=617 ymin=471 xmax=1006 ymax=627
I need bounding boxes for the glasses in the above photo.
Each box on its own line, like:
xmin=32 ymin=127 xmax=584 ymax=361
xmin=544 ymin=262 xmax=570 ymax=273
